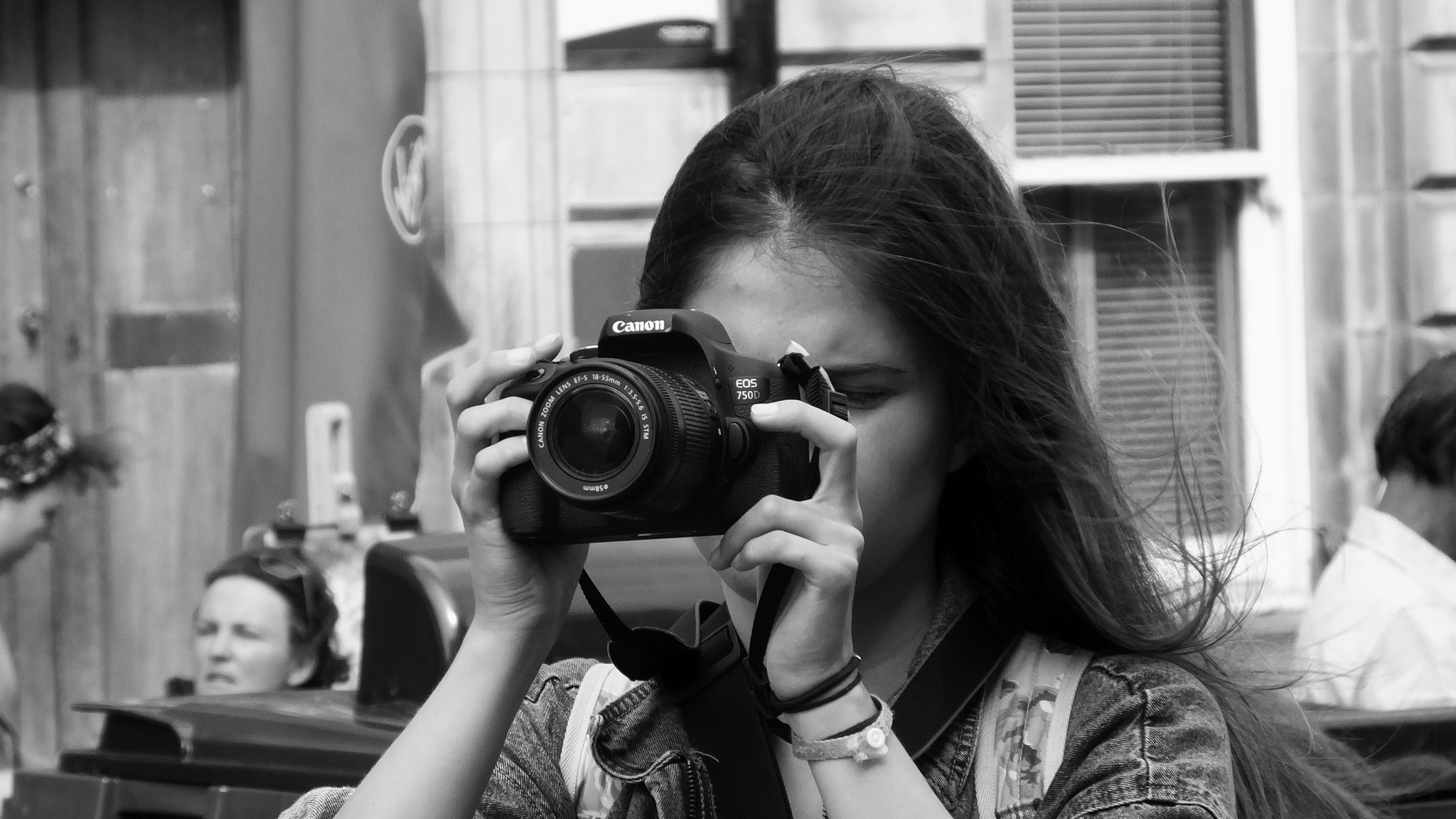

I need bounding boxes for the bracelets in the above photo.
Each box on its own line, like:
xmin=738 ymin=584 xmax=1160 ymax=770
xmin=766 ymin=656 xmax=895 ymax=760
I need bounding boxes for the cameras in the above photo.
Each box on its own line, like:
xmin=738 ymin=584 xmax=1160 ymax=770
xmin=499 ymin=307 xmax=814 ymax=548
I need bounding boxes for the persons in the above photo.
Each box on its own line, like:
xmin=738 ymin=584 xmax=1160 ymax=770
xmin=193 ymin=547 xmax=352 ymax=702
xmin=1279 ymin=352 xmax=1456 ymax=712
xmin=0 ymin=380 xmax=126 ymax=819
xmin=277 ymin=63 xmax=1421 ymax=819
